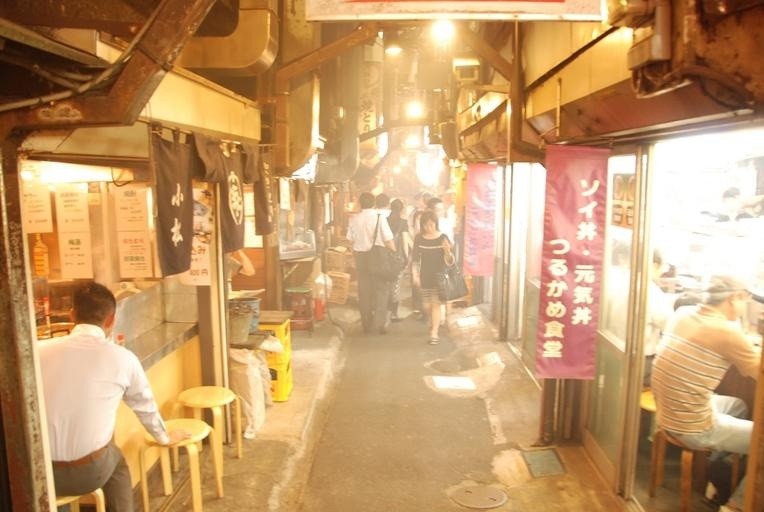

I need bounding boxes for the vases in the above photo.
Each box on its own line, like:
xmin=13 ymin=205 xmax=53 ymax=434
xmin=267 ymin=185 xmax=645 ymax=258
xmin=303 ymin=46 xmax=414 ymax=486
xmin=228 ymin=296 xmax=262 ymax=345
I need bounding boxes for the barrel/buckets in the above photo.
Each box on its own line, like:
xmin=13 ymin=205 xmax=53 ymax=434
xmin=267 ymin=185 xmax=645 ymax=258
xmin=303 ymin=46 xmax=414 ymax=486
xmin=228 ymin=310 xmax=253 ymax=345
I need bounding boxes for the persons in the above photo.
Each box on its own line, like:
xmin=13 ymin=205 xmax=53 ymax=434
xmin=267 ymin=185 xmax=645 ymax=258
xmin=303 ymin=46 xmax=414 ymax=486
xmin=650 ymin=273 xmax=754 ymax=512
xmin=348 ymin=185 xmax=459 ymax=346
xmin=602 ymin=237 xmax=675 ymax=386
xmin=38 ymin=281 xmax=193 ymax=512
xmin=722 ymin=186 xmax=743 ymax=209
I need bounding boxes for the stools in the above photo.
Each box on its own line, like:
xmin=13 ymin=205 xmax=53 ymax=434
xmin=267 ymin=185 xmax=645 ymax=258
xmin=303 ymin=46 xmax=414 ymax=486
xmin=640 ymin=389 xmax=741 ymax=511
xmin=56 ymin=386 xmax=243 ymax=512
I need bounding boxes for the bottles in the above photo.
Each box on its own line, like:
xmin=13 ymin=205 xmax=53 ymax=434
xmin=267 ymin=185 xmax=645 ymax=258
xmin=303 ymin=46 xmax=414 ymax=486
xmin=117 ymin=335 xmax=125 ymax=346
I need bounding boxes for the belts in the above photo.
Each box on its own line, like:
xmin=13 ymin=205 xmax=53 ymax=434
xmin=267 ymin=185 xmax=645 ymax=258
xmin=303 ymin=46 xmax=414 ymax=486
xmin=51 ymin=447 xmax=107 ymax=468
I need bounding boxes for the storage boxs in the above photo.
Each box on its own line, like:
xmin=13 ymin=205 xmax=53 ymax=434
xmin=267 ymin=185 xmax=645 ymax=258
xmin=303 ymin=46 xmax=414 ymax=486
xmin=323 ymin=245 xmax=351 ymax=304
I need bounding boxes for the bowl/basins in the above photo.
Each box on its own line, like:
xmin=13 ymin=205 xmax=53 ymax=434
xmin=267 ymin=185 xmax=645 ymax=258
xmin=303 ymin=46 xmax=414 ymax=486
xmin=228 ymin=296 xmax=261 ymax=345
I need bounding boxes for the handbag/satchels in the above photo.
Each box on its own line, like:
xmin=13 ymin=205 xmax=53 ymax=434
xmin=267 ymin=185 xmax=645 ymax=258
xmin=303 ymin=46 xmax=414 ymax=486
xmin=433 ymin=262 xmax=469 ymax=302
xmin=365 ymin=245 xmax=398 ymax=281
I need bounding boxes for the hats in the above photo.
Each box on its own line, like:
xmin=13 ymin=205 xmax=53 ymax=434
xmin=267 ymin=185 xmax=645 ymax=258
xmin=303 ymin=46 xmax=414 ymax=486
xmin=705 ymin=273 xmax=743 ymax=303
